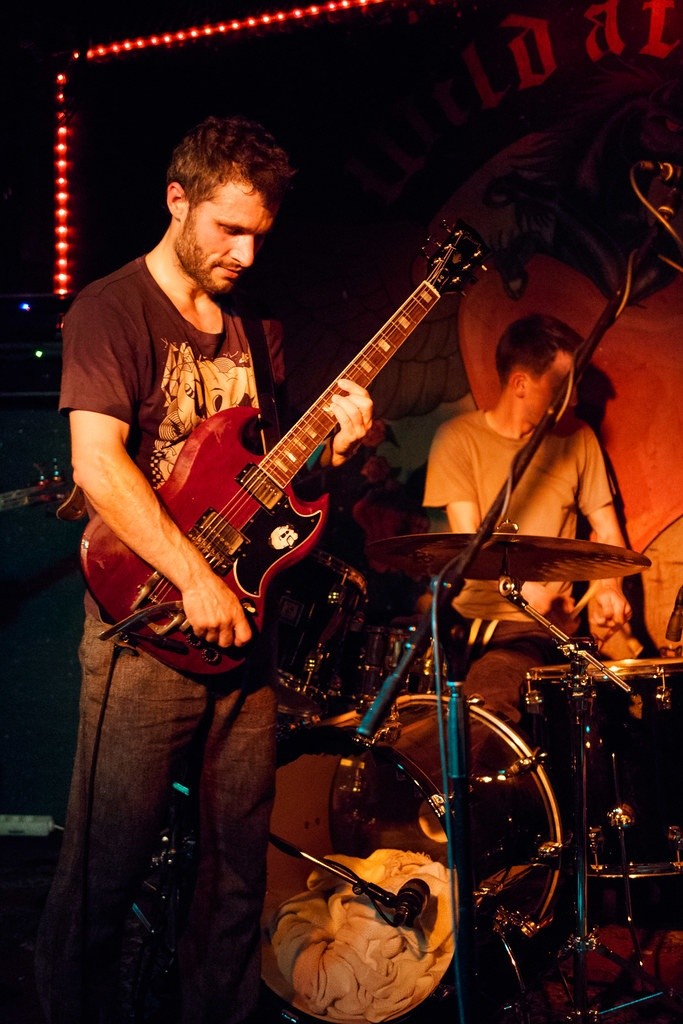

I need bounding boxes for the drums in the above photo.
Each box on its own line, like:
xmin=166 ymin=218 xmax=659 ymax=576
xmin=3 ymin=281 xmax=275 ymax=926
xmin=525 ymin=659 xmax=683 ymax=879
xmin=261 ymin=693 xmax=564 ymax=1024
xmin=348 ymin=625 xmax=448 ymax=701
xmin=243 ymin=547 xmax=369 ymax=719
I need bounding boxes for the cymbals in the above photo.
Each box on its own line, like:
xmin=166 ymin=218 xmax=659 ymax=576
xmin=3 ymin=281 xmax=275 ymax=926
xmin=364 ymin=532 xmax=652 ymax=582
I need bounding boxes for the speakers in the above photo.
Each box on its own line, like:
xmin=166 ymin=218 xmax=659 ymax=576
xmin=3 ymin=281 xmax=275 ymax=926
xmin=0 ymin=390 xmax=89 ymax=822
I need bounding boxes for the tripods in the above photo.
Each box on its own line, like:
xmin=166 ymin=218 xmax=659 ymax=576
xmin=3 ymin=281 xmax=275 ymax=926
xmin=492 ymin=567 xmax=683 ymax=1024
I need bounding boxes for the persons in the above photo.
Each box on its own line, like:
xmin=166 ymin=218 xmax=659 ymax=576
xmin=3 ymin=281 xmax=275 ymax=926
xmin=34 ymin=118 xmax=372 ymax=1024
xmin=423 ymin=314 xmax=632 ymax=782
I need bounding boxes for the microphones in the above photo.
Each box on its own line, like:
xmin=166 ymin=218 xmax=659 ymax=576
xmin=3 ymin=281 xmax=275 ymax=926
xmin=639 ymin=160 xmax=683 ymax=186
xmin=393 ymin=878 xmax=430 ymax=927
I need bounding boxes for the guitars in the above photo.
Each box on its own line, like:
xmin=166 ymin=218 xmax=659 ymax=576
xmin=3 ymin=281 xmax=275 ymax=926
xmin=0 ymin=458 xmax=71 ymax=512
xmin=79 ymin=218 xmax=488 ymax=675
